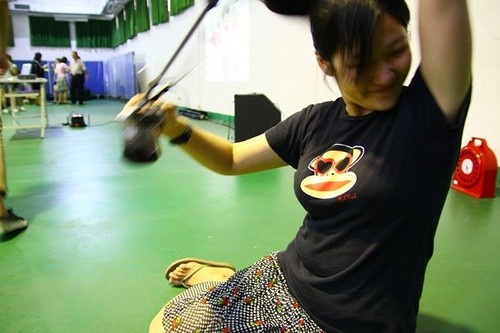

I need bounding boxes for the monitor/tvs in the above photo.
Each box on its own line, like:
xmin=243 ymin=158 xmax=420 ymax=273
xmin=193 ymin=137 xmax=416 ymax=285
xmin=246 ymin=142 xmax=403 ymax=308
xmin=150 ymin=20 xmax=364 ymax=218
xmin=21 ymin=64 xmax=32 ymax=75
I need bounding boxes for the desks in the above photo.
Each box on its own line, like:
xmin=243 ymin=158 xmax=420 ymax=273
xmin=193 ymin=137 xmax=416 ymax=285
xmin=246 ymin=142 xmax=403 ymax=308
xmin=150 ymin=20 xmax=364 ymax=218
xmin=0 ymin=78 xmax=48 ymax=137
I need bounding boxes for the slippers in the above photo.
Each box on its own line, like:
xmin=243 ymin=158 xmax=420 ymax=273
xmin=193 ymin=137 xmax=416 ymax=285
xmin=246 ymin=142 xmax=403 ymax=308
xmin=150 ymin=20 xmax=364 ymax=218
xmin=165 ymin=258 xmax=237 ymax=289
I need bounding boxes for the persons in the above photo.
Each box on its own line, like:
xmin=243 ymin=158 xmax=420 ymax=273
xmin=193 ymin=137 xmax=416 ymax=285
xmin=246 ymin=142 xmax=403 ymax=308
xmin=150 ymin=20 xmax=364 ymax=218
xmin=0 ymin=50 xmax=88 ymax=114
xmin=0 ymin=0 xmax=28 ymax=238
xmin=123 ymin=0 xmax=474 ymax=333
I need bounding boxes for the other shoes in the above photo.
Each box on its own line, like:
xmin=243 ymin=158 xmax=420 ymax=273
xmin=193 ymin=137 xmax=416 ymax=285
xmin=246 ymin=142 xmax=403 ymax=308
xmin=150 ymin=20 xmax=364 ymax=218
xmin=0 ymin=209 xmax=28 ymax=242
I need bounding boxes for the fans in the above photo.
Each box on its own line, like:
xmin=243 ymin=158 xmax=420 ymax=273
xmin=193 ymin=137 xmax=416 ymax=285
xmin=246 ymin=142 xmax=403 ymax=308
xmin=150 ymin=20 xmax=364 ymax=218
xmin=450 ymin=136 xmax=497 ymax=199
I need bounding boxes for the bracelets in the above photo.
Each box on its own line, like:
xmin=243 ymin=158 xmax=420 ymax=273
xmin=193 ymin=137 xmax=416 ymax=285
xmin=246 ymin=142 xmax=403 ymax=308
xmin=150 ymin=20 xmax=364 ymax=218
xmin=169 ymin=123 xmax=192 ymax=145
